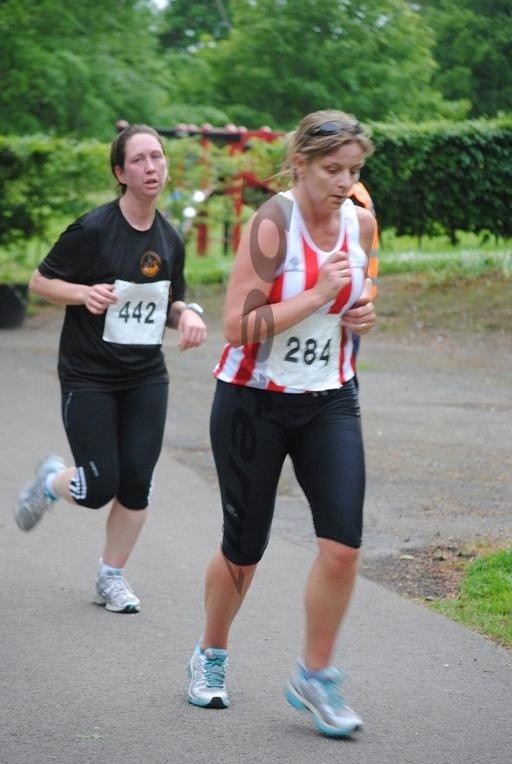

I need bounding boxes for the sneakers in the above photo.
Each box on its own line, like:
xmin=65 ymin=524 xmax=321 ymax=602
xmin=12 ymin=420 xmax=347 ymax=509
xmin=284 ymin=659 xmax=363 ymax=737
xmin=13 ymin=455 xmax=67 ymax=531
xmin=184 ymin=634 xmax=230 ymax=709
xmin=92 ymin=569 xmax=141 ymax=613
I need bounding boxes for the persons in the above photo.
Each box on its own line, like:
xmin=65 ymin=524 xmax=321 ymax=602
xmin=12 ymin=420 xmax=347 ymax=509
xmin=13 ymin=121 xmax=208 ymax=610
xmin=183 ymin=109 xmax=375 ymax=736
xmin=348 ymin=183 xmax=382 ymax=390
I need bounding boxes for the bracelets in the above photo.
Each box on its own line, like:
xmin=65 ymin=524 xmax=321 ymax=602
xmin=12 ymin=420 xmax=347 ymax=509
xmin=177 ymin=301 xmax=203 ymax=315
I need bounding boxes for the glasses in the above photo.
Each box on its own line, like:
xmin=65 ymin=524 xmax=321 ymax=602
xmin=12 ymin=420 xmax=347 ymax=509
xmin=308 ymin=120 xmax=363 ymax=135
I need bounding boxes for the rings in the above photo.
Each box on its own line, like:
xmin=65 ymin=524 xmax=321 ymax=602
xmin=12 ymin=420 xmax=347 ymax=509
xmin=362 ymin=321 xmax=366 ymax=330
